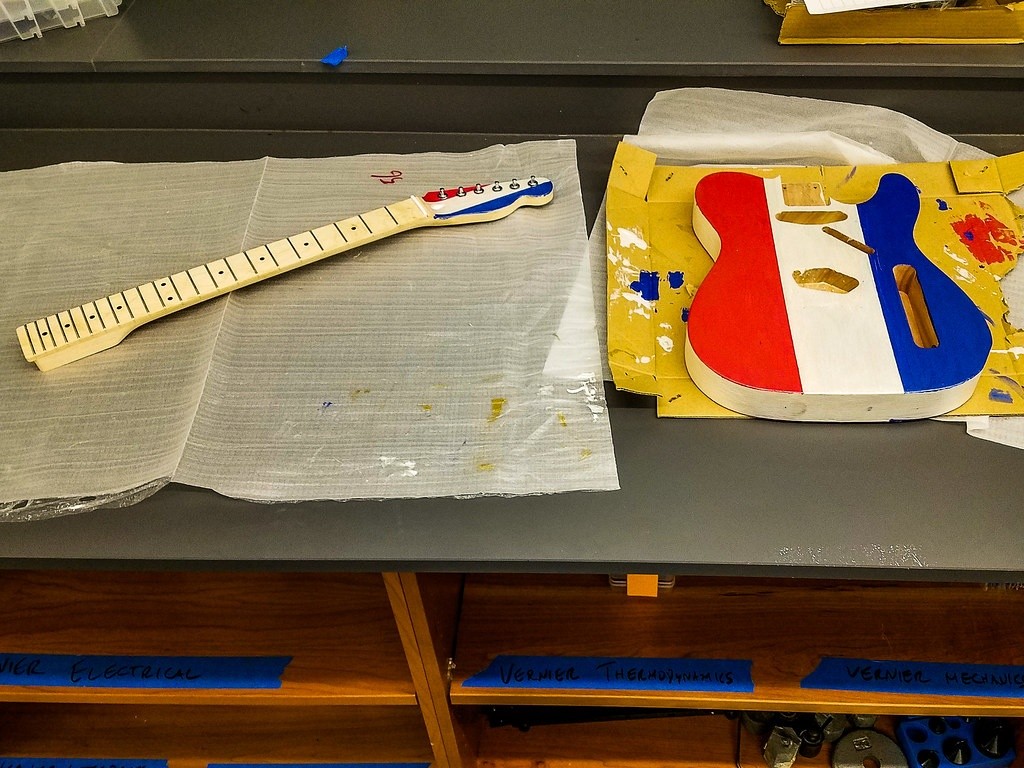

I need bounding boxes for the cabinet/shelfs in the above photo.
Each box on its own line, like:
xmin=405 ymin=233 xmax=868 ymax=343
xmin=0 ymin=0 xmax=1023 ymax=410
xmin=1 ymin=411 xmax=1024 ymax=768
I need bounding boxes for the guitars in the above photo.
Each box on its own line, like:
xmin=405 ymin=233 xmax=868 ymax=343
xmin=11 ymin=170 xmax=555 ymax=380
xmin=685 ymin=166 xmax=993 ymax=431
